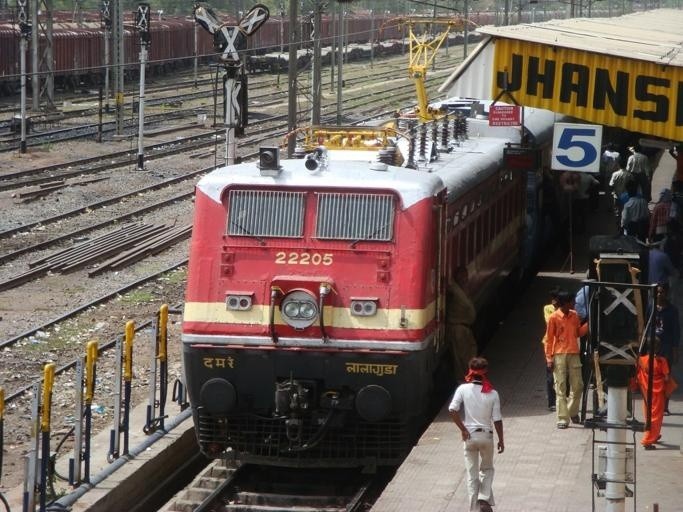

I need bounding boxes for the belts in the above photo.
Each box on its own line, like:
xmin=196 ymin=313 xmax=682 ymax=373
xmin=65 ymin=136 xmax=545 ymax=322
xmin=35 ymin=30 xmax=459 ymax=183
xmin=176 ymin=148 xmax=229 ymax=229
xmin=476 ymin=428 xmax=493 ymax=433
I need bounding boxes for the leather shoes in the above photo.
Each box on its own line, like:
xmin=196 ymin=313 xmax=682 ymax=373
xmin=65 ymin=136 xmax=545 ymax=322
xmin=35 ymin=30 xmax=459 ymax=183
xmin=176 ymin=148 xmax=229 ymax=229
xmin=557 ymin=423 xmax=567 ymax=429
xmin=479 ymin=501 xmax=493 ymax=511
xmin=570 ymin=416 xmax=580 ymax=424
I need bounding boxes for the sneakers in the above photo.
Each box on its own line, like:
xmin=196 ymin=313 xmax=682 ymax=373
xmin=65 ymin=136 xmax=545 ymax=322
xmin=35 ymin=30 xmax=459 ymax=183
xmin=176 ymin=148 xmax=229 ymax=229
xmin=550 ymin=405 xmax=556 ymax=411
xmin=643 ymin=445 xmax=655 ymax=450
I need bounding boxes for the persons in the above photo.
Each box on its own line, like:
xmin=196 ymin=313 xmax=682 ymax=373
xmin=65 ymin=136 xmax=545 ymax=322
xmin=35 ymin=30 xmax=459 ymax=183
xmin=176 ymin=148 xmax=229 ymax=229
xmin=540 ymin=287 xmax=590 ymax=429
xmin=544 ymin=134 xmax=682 ymax=288
xmin=447 ymin=356 xmax=504 ymax=511
xmin=446 ymin=262 xmax=480 ymax=387
xmin=573 ymin=277 xmax=591 ymax=355
xmin=538 ymin=284 xmax=565 ymax=411
xmin=645 ymin=279 xmax=682 ymax=417
xmin=629 ymin=333 xmax=679 ymax=451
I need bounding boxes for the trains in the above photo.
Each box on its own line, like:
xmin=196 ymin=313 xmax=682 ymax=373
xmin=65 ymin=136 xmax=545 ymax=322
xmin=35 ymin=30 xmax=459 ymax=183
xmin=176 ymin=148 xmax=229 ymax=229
xmin=180 ymin=84 xmax=594 ymax=474
xmin=0 ymin=4 xmax=640 ymax=100
